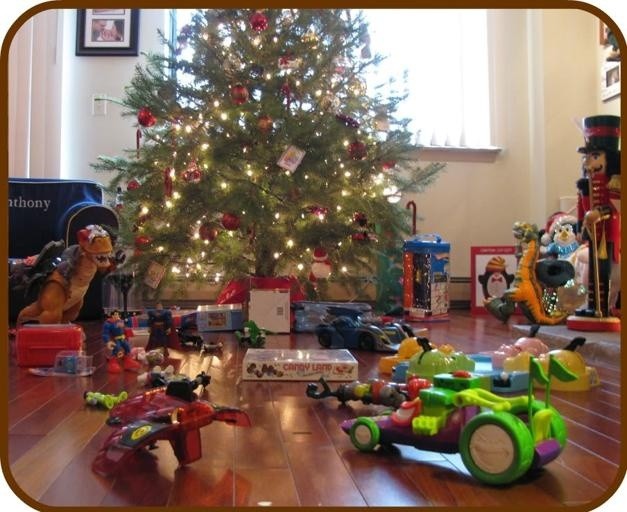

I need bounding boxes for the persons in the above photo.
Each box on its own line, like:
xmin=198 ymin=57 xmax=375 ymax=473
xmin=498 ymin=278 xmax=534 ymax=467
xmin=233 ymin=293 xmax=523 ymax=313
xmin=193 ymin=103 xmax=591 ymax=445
xmin=84 ymin=389 xmax=128 ymax=411
xmin=91 ymin=19 xmax=124 ymax=42
xmin=146 ymin=301 xmax=173 ymax=359
xmin=102 ymin=309 xmax=147 ymax=372
xmin=137 ymin=366 xmax=178 ymax=387
xmin=571 ymin=115 xmax=621 ymax=318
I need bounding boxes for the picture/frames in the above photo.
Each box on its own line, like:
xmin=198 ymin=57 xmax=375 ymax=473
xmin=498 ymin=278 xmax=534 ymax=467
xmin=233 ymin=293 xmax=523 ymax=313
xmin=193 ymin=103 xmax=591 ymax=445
xmin=74 ymin=9 xmax=140 ymax=57
